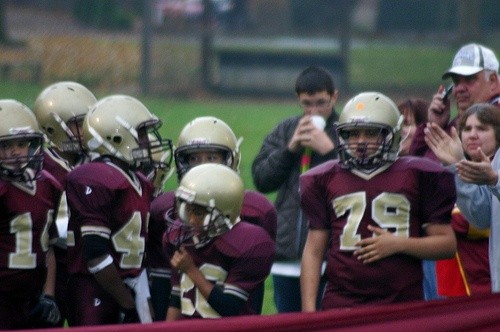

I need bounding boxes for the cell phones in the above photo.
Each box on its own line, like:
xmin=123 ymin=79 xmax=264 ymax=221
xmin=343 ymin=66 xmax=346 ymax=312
xmin=434 ymin=84 xmax=453 ymax=114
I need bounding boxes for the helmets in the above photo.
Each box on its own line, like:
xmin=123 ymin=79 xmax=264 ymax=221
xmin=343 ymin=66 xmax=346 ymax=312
xmin=0 ymin=100 xmax=43 ymax=141
xmin=334 ymin=91 xmax=401 ymax=128
xmin=174 ymin=162 xmax=244 ymax=226
xmin=34 ymin=81 xmax=98 ymax=152
xmin=174 ymin=116 xmax=241 ymax=172
xmin=83 ymin=94 xmax=159 ymax=163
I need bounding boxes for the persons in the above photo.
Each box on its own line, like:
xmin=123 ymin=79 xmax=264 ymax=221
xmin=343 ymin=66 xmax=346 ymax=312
xmin=424 ymin=122 xmax=500 ymax=294
xmin=64 ymin=93 xmax=173 ymax=328
xmin=300 ymin=91 xmax=457 ymax=313
xmin=397 ymin=42 xmax=500 ymax=155
xmin=161 ymin=164 xmax=276 ymax=322
xmin=33 ymin=83 xmax=98 ymax=167
xmin=145 ymin=116 xmax=274 ymax=323
xmin=251 ymin=68 xmax=342 ymax=313
xmin=0 ymin=100 xmax=62 ymax=329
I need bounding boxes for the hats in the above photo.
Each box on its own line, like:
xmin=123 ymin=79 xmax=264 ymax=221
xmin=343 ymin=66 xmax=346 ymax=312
xmin=441 ymin=43 xmax=499 ymax=80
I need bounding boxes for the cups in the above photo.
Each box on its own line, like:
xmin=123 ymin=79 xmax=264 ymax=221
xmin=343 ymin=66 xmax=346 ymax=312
xmin=311 ymin=115 xmax=325 ymax=131
